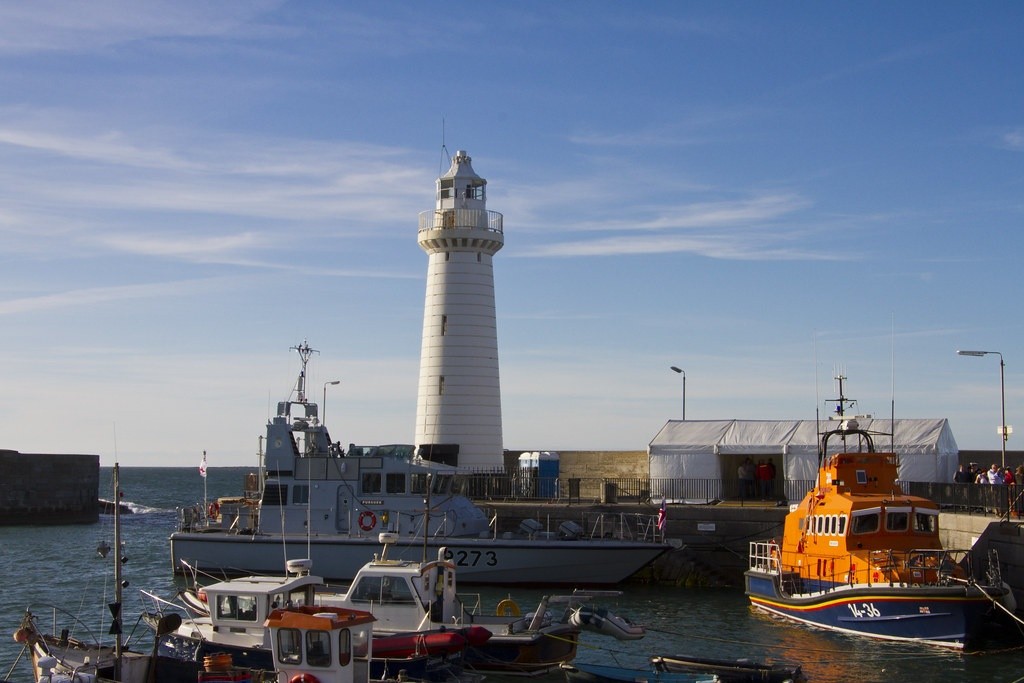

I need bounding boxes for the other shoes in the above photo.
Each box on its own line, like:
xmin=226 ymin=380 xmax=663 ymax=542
xmin=760 ymin=498 xmax=766 ymax=501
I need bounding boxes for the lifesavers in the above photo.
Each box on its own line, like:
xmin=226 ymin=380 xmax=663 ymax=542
xmin=289 ymin=673 xmax=320 ymax=683
xmin=208 ymin=502 xmax=220 ymax=519
xmin=797 ymin=539 xmax=805 ymax=552
xmin=497 ymin=600 xmax=520 ymax=617
xmin=358 ymin=511 xmax=376 ymax=532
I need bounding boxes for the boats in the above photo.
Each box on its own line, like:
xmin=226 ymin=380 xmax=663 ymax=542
xmin=649 ymin=652 xmax=807 ymax=683
xmin=740 ymin=310 xmax=1018 ymax=655
xmin=14 ymin=459 xmax=723 ymax=683
xmin=169 ymin=334 xmax=673 ymax=594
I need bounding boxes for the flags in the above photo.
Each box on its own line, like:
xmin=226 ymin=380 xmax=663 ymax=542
xmin=659 ymin=499 xmax=667 ymax=532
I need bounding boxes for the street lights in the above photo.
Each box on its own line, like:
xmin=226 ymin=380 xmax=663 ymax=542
xmin=670 ymin=365 xmax=687 ymax=422
xmin=321 ymin=379 xmax=340 ymax=425
xmin=956 ymin=350 xmax=1006 ymax=468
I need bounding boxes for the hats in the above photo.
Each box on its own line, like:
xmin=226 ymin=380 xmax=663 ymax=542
xmin=745 ymin=457 xmax=752 ymax=464
xmin=970 ymin=463 xmax=978 ymax=467
xmin=1006 ymin=466 xmax=1011 ymax=469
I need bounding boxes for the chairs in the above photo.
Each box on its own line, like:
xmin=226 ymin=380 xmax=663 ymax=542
xmin=313 ymin=641 xmax=323 ymax=656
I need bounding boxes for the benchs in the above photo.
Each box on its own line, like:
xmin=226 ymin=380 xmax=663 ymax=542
xmin=635 ymin=678 xmax=649 ymax=683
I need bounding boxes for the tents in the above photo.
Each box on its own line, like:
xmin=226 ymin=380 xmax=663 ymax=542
xmin=647 ymin=417 xmax=960 ymax=506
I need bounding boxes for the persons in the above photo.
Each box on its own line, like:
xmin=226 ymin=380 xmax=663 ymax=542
xmin=735 ymin=457 xmax=777 ymax=502
xmin=954 ymin=462 xmax=1024 ymax=518
